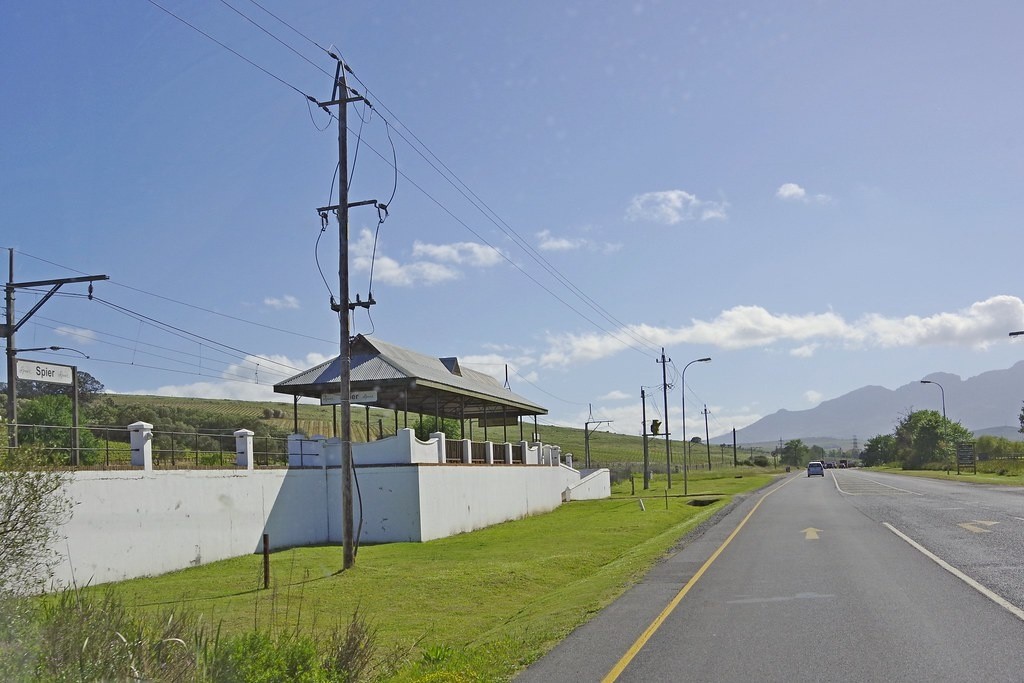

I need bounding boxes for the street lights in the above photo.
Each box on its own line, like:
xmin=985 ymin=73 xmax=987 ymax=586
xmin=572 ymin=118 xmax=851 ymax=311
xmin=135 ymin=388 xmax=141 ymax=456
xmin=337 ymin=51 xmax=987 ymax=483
xmin=682 ymin=356 xmax=713 ymax=495
xmin=921 ymin=380 xmax=950 ymax=474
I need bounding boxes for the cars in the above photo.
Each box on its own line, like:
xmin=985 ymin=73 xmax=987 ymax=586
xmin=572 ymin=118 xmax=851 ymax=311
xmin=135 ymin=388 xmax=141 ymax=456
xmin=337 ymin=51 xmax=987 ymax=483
xmin=806 ymin=461 xmax=825 ymax=477
xmin=818 ymin=459 xmax=867 ymax=469
